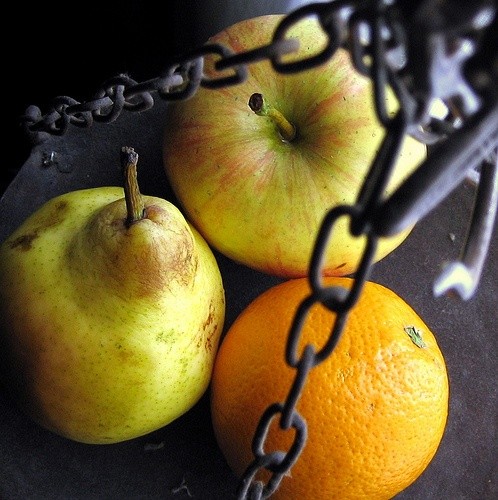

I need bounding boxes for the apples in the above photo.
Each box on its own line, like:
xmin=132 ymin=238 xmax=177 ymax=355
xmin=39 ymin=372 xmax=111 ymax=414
xmin=158 ymin=14 xmax=429 ymax=281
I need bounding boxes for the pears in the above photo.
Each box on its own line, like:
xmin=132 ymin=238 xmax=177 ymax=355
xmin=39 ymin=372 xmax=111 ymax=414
xmin=0 ymin=142 xmax=226 ymax=444
